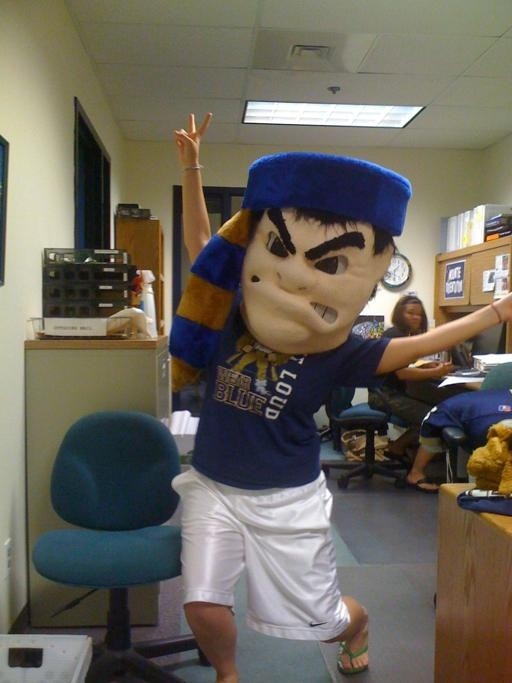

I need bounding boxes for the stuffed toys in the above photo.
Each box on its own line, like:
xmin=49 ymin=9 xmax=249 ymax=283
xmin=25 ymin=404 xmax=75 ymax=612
xmin=468 ymin=419 xmax=512 ymax=497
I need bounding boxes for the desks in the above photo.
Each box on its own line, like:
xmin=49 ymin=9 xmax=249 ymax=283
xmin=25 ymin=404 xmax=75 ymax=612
xmin=407 ymin=235 xmax=512 ymax=397
xmin=0 ymin=633 xmax=93 ymax=683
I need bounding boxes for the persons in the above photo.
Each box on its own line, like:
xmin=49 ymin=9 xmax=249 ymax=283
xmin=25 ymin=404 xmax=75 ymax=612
xmin=171 ymin=112 xmax=512 ymax=681
xmin=368 ymin=293 xmax=454 ymax=493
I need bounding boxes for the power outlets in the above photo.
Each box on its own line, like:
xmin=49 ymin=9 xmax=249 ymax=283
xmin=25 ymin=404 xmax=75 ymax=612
xmin=4 ymin=538 xmax=13 ymax=579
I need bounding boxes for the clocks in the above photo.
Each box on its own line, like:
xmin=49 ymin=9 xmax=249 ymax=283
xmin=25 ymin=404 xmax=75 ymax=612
xmin=381 ymin=253 xmax=411 ymax=288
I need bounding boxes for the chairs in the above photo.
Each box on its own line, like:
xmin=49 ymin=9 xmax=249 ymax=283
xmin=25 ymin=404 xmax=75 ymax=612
xmin=32 ymin=410 xmax=211 ymax=682
xmin=320 ymin=388 xmax=412 ymax=490
xmin=442 ymin=362 xmax=512 ymax=483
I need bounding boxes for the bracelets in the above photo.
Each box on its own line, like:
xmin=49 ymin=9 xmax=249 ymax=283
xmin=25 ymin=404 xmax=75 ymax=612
xmin=181 ymin=164 xmax=203 ymax=170
xmin=491 ymin=302 xmax=501 ymax=324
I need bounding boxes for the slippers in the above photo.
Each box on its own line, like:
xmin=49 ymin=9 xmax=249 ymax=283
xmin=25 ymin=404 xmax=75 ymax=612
xmin=407 ymin=478 xmax=438 ymax=494
xmin=336 ymin=641 xmax=368 ymax=675
xmin=384 ymin=448 xmax=413 ymax=468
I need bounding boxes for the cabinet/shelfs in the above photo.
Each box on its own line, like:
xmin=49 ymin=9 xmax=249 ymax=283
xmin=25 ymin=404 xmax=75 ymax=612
xmin=115 ymin=217 xmax=165 ymax=335
xmin=435 ymin=483 xmax=512 ymax=683
xmin=24 ymin=336 xmax=171 ymax=628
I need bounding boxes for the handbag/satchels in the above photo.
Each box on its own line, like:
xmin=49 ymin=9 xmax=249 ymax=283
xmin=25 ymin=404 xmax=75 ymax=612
xmin=341 ymin=430 xmax=392 ymax=463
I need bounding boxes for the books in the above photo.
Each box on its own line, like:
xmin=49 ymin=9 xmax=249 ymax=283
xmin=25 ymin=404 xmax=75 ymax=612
xmin=485 ymin=209 xmax=511 ymax=240
